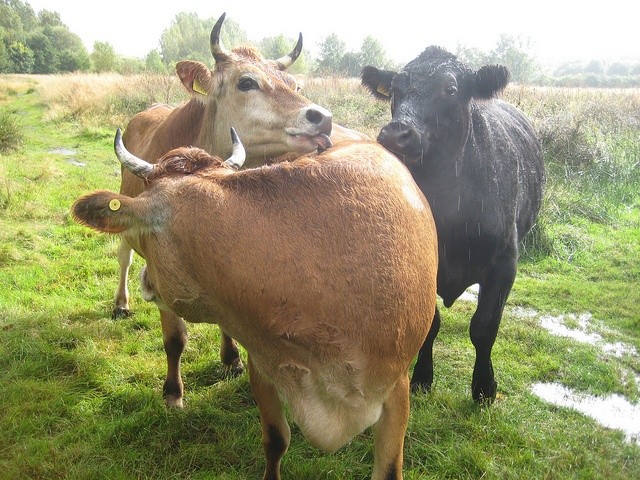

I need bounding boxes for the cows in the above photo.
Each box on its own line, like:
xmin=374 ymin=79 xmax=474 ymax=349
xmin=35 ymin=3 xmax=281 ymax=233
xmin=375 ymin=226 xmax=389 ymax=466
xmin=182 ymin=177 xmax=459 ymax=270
xmin=110 ymin=11 xmax=334 ymax=417
xmin=360 ymin=46 xmax=544 ymax=407
xmin=73 ymin=126 xmax=439 ymax=480
